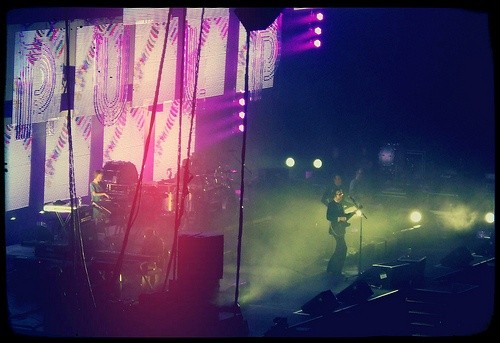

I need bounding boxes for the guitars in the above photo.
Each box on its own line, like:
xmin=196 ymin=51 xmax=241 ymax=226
xmin=344 ymin=205 xmax=352 ymax=211
xmin=330 ymin=205 xmax=363 ymax=237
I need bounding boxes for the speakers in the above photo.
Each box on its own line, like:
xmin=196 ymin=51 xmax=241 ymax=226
xmin=440 ymin=245 xmax=475 ymax=268
xmin=301 ymin=289 xmax=339 ymax=315
xmin=336 ymin=277 xmax=375 ymax=305
xmin=392 ymin=262 xmax=432 ymax=289
xmin=358 ymin=263 xmax=393 ymax=290
xmin=178 ymin=231 xmax=224 ymax=280
xmin=168 ymin=279 xmax=220 ymax=329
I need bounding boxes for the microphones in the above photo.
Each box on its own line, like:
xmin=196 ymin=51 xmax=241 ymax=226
xmin=348 ymin=195 xmax=354 ymax=201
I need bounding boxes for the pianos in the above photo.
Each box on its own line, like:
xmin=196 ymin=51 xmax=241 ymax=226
xmin=42 ymin=202 xmax=90 ymax=235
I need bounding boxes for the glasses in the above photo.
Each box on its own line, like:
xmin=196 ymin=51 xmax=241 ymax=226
xmin=336 ymin=192 xmax=343 ymax=196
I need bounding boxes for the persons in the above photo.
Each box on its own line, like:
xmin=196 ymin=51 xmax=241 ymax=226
xmin=90 ymin=169 xmax=111 ymax=224
xmin=350 ymin=169 xmax=365 ymax=199
xmin=321 ymin=176 xmax=353 ymax=207
xmin=327 ymin=189 xmax=358 ymax=277
